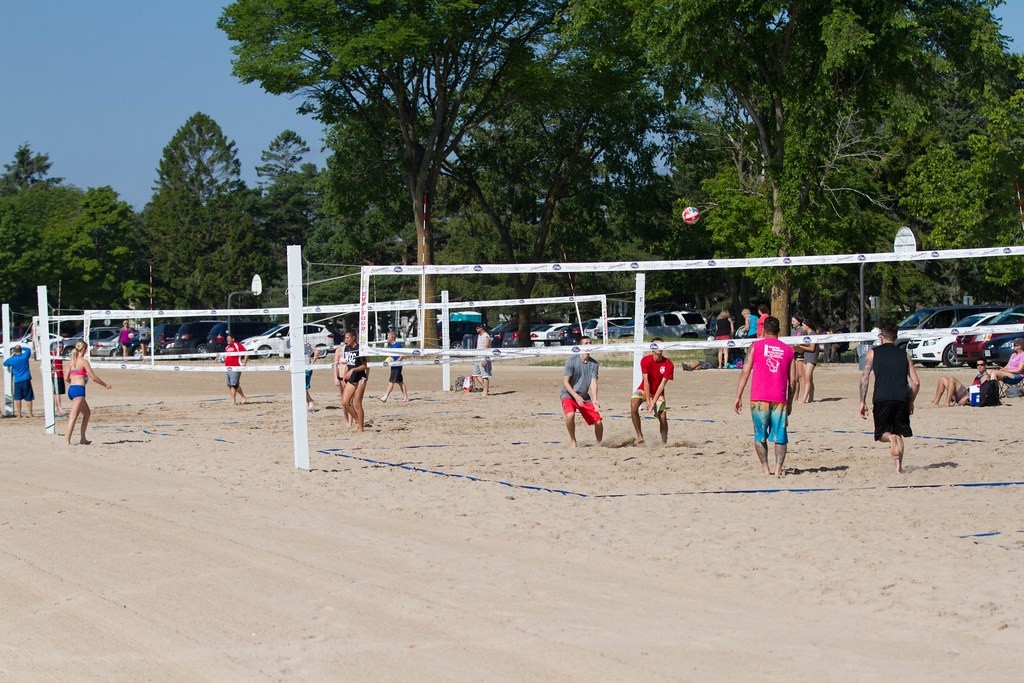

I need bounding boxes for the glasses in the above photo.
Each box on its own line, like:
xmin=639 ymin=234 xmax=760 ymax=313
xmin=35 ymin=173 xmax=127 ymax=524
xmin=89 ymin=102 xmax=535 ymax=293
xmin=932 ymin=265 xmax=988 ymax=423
xmin=1014 ymin=343 xmax=1020 ymax=346
xmin=877 ymin=333 xmax=884 ymax=338
xmin=976 ymin=364 xmax=985 ymax=366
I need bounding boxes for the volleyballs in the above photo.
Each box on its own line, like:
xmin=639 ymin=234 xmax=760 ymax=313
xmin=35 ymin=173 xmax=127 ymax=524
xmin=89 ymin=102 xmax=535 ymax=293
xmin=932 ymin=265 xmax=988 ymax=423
xmin=682 ymin=206 xmax=699 ymax=225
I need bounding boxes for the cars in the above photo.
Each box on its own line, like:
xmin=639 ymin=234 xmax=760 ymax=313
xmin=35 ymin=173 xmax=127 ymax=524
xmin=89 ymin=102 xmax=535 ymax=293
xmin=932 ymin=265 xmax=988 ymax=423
xmin=412 ymin=310 xmax=710 ymax=350
xmin=873 ymin=304 xmax=1024 ymax=369
xmin=0 ymin=333 xmax=70 ymax=358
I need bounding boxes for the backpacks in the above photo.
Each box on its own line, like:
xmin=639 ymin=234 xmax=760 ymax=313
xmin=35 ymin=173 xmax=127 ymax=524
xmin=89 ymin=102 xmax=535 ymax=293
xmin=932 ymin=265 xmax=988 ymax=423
xmin=455 ymin=375 xmax=465 ymax=391
xmin=979 ymin=380 xmax=1003 ymax=407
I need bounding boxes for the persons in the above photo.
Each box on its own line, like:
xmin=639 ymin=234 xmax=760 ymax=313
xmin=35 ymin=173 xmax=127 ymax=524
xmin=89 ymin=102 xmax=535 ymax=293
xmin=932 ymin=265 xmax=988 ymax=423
xmin=65 ymin=340 xmax=111 ymax=444
xmin=377 ymin=332 xmax=409 ymax=402
xmin=682 ymin=336 xmax=718 ymax=372
xmin=304 ymin=342 xmax=319 ymax=410
xmin=630 ymin=337 xmax=674 ymax=444
xmin=859 ymin=322 xmax=920 ymax=473
xmin=215 ymin=334 xmax=248 ymax=405
xmin=734 ymin=317 xmax=796 ymax=476
xmin=708 ymin=304 xmax=770 ymax=369
xmin=334 ymin=329 xmax=371 ymax=432
xmin=560 ymin=336 xmax=604 ymax=447
xmin=138 ymin=321 xmax=151 ymax=356
xmin=50 ymin=341 xmax=66 ymax=417
xmin=990 ymin=338 xmax=1024 ymax=385
xmin=933 ymin=357 xmax=990 ymax=406
xmin=472 ymin=323 xmax=492 ymax=398
xmin=816 ymin=320 xmax=850 ymax=362
xmin=118 ymin=320 xmax=134 ymax=357
xmin=3 ymin=345 xmax=35 ymax=418
xmin=791 ymin=314 xmax=818 ymax=403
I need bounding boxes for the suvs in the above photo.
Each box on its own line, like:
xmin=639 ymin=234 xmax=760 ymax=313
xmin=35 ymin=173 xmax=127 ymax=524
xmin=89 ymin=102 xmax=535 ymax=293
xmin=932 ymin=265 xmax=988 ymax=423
xmin=239 ymin=322 xmax=335 ymax=359
xmin=51 ymin=319 xmax=282 ymax=361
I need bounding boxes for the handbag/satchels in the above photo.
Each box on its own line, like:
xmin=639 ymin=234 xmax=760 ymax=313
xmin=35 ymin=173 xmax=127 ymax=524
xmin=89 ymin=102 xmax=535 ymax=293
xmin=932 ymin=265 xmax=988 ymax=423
xmin=463 ymin=376 xmax=475 ymax=392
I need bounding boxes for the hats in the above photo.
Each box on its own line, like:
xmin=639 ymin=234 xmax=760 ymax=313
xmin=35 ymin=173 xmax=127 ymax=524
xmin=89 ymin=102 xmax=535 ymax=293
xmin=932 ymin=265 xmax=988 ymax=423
xmin=1010 ymin=337 xmax=1024 ymax=345
